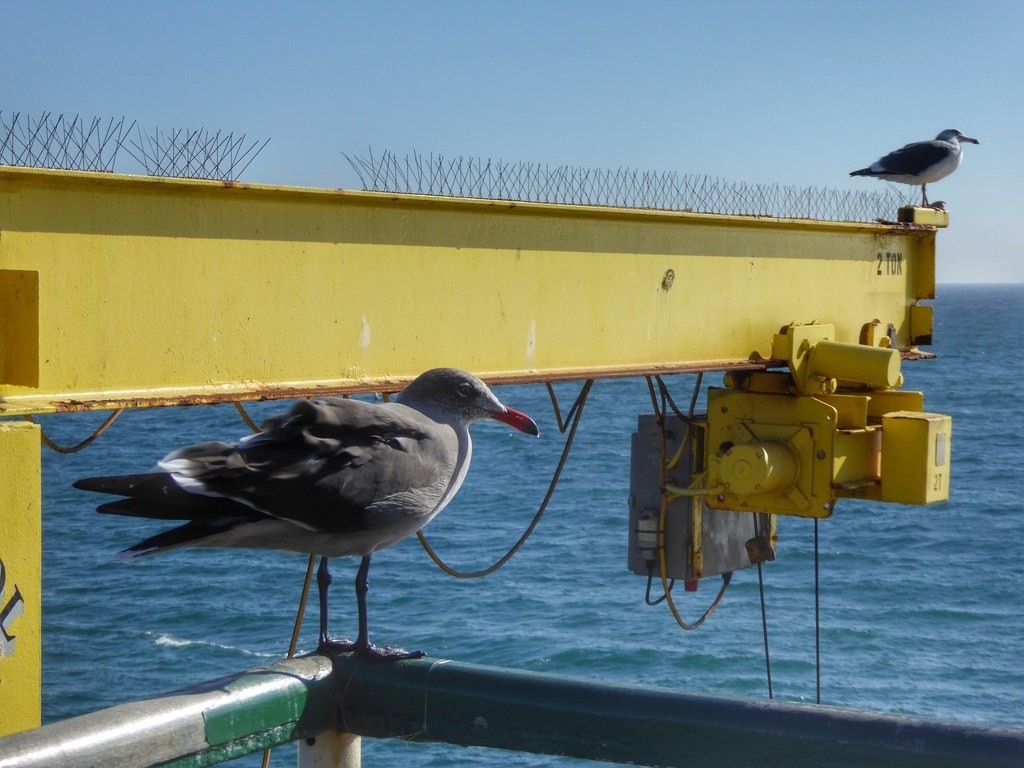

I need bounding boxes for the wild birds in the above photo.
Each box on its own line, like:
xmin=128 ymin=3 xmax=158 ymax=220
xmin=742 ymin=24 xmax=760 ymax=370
xmin=848 ymin=129 xmax=980 ymax=213
xmin=74 ymin=369 xmax=540 ymax=662
